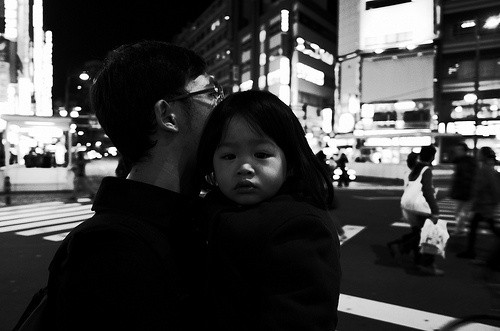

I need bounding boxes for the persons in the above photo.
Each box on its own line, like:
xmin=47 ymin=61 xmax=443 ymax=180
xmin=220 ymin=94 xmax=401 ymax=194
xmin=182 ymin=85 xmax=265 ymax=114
xmin=388 ymin=146 xmax=446 ymax=276
xmin=449 ymin=144 xmax=500 ymax=259
xmin=63 ymin=151 xmax=97 ymax=204
xmin=42 ymin=41 xmax=220 ymax=331
xmin=197 ymin=91 xmax=341 ymax=331
xmin=335 ymin=154 xmax=349 ymax=186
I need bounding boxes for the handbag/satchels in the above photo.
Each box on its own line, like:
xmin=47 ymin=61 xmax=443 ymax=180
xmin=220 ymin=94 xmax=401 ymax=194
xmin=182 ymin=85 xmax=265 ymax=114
xmin=419 ymin=218 xmax=450 ymax=251
xmin=401 ymin=166 xmax=439 ymax=214
xmin=420 ymin=246 xmax=446 ymax=258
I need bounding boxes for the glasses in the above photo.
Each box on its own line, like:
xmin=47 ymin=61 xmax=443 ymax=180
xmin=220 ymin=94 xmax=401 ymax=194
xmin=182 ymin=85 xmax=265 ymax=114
xmin=167 ymin=76 xmax=225 ymax=102
